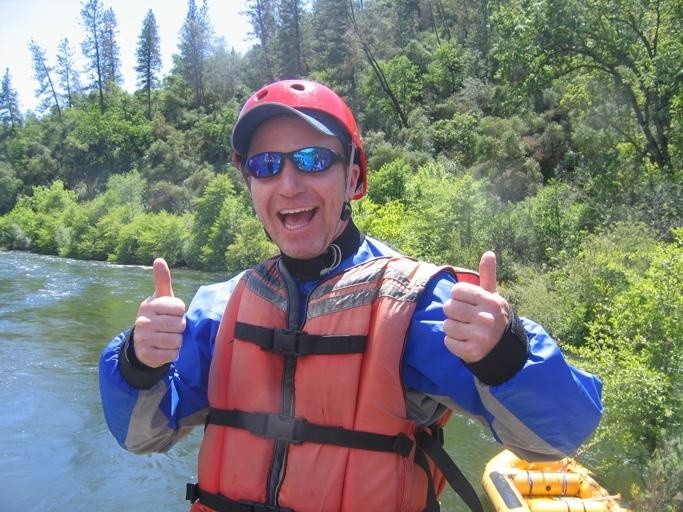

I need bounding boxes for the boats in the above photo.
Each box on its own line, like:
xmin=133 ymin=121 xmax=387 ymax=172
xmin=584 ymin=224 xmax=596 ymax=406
xmin=481 ymin=449 xmax=627 ymax=512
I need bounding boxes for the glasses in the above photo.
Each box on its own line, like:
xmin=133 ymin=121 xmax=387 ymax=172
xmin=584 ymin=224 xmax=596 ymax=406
xmin=242 ymin=146 xmax=350 ymax=179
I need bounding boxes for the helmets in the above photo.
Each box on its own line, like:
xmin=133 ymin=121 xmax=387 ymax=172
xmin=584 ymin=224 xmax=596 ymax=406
xmin=231 ymin=78 xmax=367 ymax=200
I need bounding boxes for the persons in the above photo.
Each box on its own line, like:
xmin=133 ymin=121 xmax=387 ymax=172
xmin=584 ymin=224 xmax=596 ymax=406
xmin=98 ymin=80 xmax=603 ymax=512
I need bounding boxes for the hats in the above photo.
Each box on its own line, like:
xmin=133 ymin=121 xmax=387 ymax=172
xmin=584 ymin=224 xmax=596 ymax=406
xmin=231 ymin=102 xmax=349 ymax=157
